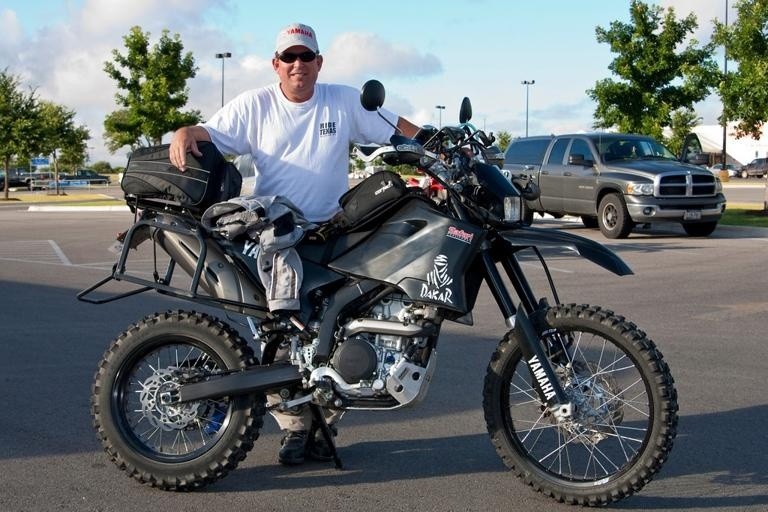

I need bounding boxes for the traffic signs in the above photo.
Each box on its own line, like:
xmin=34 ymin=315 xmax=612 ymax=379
xmin=30 ymin=159 xmax=50 ymax=165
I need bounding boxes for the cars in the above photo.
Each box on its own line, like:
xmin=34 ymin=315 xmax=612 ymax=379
xmin=706 ymin=163 xmax=741 ymax=177
xmin=0 ymin=167 xmax=51 ymax=192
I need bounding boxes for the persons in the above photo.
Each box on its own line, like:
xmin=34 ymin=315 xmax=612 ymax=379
xmin=168 ymin=24 xmax=476 ymax=466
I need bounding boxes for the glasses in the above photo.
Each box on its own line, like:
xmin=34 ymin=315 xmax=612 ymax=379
xmin=276 ymin=53 xmax=316 ymax=62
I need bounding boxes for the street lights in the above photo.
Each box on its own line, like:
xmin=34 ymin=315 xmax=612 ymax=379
xmin=435 ymin=105 xmax=447 ymax=132
xmin=215 ymin=52 xmax=233 ymax=108
xmin=521 ymin=78 xmax=536 ymax=138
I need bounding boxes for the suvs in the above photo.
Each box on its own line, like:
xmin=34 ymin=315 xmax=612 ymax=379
xmin=736 ymin=156 xmax=767 ymax=179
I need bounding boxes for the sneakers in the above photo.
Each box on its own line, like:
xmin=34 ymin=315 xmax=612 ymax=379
xmin=307 ymin=422 xmax=338 ymax=462
xmin=278 ymin=430 xmax=309 ymax=464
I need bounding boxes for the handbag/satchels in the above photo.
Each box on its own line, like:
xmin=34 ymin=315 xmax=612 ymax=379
xmin=121 ymin=139 xmax=241 ymax=212
xmin=338 ymin=171 xmax=405 ymax=228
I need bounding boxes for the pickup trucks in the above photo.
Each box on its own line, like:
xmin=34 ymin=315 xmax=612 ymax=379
xmin=58 ymin=168 xmax=114 ymax=186
xmin=496 ymin=132 xmax=727 ymax=242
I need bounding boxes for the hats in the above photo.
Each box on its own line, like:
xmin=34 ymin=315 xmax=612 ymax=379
xmin=277 ymin=23 xmax=317 ymax=55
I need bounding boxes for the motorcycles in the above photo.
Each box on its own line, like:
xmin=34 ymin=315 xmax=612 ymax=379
xmin=73 ymin=76 xmax=679 ymax=508
xmin=391 ymin=143 xmax=492 ymax=231
